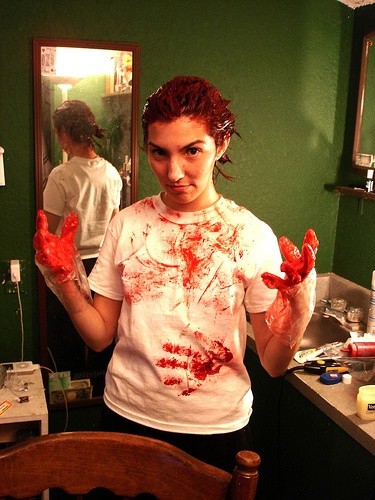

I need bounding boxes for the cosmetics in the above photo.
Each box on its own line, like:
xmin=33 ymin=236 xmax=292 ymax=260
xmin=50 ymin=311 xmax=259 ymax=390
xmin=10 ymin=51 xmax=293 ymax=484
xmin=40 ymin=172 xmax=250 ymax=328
xmin=356 ymin=384 xmax=375 ymax=420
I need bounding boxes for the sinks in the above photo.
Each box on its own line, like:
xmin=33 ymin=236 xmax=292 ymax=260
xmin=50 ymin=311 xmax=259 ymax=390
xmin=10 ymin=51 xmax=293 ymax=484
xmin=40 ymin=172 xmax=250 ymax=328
xmin=245 ymin=272 xmax=364 ymax=364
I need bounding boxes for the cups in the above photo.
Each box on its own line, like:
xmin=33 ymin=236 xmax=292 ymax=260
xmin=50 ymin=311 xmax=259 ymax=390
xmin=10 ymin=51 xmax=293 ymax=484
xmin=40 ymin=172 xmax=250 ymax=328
xmin=356 ymin=153 xmax=373 ymax=167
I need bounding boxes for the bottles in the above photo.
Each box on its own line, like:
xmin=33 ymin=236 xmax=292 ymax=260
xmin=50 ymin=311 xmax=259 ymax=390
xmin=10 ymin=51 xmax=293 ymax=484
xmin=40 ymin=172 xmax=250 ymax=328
xmin=340 ymin=342 xmax=375 ymax=356
xmin=365 ymin=270 xmax=375 ymax=336
xmin=356 ymin=385 xmax=375 ymax=422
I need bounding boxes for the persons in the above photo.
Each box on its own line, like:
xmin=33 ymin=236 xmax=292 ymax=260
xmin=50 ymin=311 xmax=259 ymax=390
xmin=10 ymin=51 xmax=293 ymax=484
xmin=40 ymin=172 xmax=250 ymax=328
xmin=35 ymin=77 xmax=320 ymax=475
xmin=41 ymin=99 xmax=122 ymax=395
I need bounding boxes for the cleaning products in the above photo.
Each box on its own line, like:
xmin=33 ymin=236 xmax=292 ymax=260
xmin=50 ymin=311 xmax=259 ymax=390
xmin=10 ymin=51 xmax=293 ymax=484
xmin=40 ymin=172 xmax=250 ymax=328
xmin=365 ymin=271 xmax=375 ymax=335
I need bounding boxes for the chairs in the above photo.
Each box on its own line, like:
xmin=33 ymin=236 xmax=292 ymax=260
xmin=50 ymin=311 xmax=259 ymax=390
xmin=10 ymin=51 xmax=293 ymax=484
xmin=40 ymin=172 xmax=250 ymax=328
xmin=10 ymin=260 xmax=21 ymax=282
xmin=0 ymin=431 xmax=262 ymax=500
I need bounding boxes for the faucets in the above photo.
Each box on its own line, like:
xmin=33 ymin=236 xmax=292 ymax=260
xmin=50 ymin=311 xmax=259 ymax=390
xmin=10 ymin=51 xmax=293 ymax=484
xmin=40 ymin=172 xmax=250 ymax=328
xmin=319 ymin=291 xmax=363 ymax=324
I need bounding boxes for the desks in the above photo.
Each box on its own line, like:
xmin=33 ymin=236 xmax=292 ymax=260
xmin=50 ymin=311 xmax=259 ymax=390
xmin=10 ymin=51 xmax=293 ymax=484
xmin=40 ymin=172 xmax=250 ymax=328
xmin=0 ymin=361 xmax=48 ymax=436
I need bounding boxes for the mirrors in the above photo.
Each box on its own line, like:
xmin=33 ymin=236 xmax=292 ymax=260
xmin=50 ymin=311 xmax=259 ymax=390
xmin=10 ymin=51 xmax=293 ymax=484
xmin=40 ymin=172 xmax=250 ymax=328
xmin=34 ymin=37 xmax=140 ymax=264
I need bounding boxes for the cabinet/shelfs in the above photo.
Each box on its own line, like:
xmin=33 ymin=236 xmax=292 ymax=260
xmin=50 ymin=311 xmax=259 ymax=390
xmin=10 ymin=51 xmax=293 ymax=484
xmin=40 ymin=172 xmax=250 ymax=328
xmin=247 ymin=272 xmax=375 ymax=500
